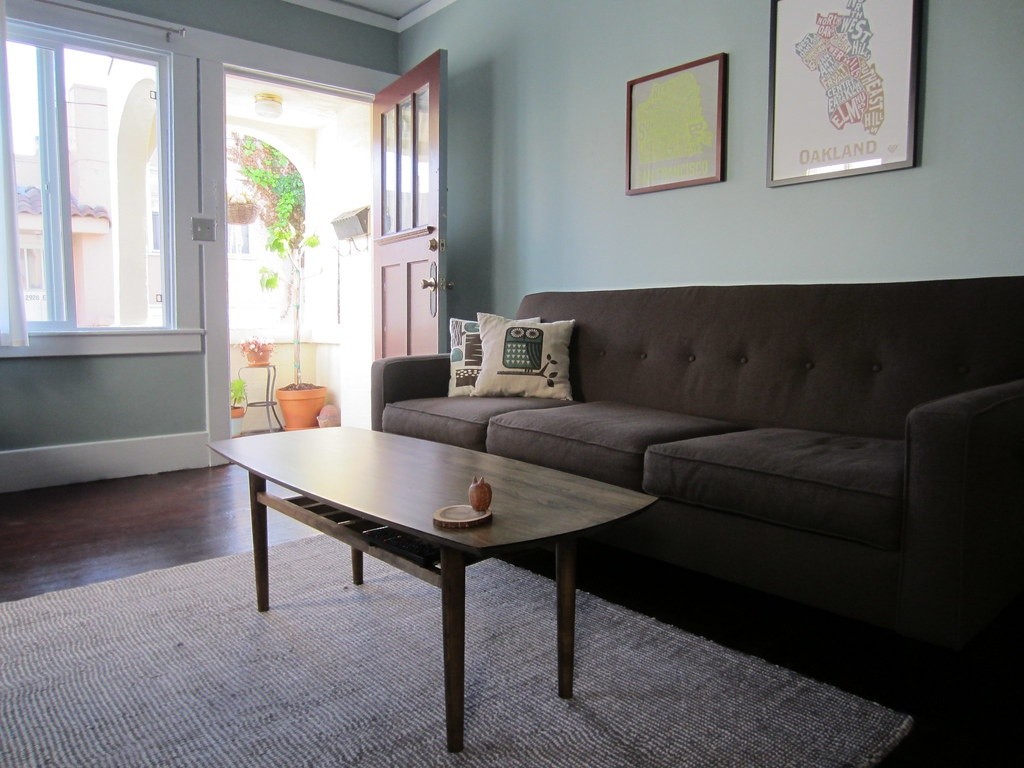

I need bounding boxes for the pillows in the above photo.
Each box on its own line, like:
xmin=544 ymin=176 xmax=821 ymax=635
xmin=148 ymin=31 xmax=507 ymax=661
xmin=470 ymin=312 xmax=575 ymax=402
xmin=448 ymin=317 xmax=541 ymax=398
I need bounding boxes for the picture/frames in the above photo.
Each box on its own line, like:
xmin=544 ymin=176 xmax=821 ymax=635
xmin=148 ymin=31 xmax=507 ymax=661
xmin=625 ymin=53 xmax=729 ymax=195
xmin=765 ymin=0 xmax=923 ymax=189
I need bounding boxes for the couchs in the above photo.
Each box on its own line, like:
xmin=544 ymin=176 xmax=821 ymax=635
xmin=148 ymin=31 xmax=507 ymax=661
xmin=371 ymin=275 xmax=1024 ymax=651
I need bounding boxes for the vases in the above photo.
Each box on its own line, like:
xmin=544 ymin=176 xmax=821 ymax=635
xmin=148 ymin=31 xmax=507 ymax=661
xmin=246 ymin=345 xmax=272 ymax=366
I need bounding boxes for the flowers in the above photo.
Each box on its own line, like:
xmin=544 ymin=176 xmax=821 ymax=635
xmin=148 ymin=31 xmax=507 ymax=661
xmin=239 ymin=335 xmax=277 ymax=358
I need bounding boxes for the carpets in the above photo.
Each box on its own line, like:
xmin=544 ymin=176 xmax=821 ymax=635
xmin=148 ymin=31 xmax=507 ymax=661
xmin=0 ymin=534 xmax=914 ymax=768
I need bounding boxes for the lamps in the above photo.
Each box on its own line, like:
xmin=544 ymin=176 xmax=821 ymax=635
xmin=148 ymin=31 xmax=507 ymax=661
xmin=255 ymin=93 xmax=283 ymax=118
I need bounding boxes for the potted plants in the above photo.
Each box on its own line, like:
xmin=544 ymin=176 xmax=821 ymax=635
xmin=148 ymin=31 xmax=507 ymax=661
xmin=230 ymin=379 xmax=248 ymax=418
xmin=259 ymin=232 xmax=326 ymax=431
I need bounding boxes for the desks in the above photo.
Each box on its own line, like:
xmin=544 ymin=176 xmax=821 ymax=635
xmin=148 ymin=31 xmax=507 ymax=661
xmin=206 ymin=425 xmax=658 ymax=753
